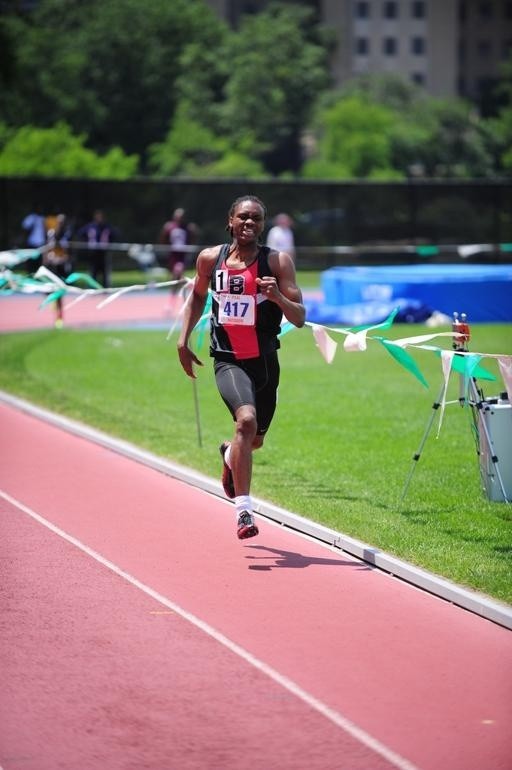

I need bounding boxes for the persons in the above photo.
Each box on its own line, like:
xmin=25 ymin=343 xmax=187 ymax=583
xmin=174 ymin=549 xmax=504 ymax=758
xmin=178 ymin=195 xmax=306 ymax=539
xmin=156 ymin=207 xmax=202 ymax=311
xmin=266 ymin=212 xmax=297 ymax=261
xmin=20 ymin=204 xmax=74 ymax=330
xmin=77 ymin=208 xmax=124 ymax=294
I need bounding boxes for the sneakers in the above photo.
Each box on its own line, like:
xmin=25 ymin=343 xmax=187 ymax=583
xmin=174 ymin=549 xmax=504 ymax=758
xmin=235 ymin=509 xmax=259 ymax=539
xmin=219 ymin=441 xmax=235 ymax=498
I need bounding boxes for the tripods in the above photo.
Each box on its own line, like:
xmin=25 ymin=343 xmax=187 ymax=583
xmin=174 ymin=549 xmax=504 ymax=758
xmin=395 ymin=343 xmax=512 ymax=518
